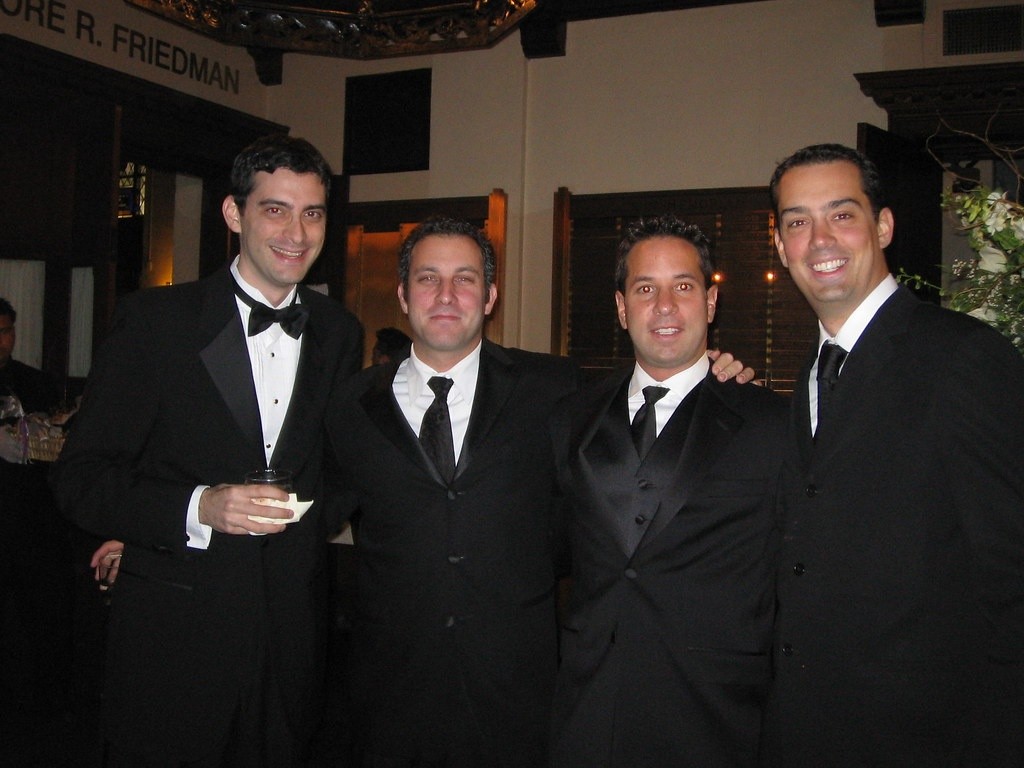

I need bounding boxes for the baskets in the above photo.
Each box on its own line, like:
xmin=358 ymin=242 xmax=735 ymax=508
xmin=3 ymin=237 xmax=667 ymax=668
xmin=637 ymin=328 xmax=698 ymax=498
xmin=4 ymin=391 xmax=66 ymax=462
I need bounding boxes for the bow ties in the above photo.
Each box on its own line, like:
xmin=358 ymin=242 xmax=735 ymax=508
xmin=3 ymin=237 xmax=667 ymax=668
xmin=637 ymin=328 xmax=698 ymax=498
xmin=228 ymin=270 xmax=308 ymax=340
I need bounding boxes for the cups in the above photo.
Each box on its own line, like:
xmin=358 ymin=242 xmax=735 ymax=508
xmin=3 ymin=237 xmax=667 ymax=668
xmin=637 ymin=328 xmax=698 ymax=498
xmin=245 ymin=468 xmax=294 ymax=494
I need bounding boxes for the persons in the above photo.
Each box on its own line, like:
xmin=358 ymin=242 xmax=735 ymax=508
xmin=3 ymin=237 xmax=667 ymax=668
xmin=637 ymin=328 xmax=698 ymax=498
xmin=767 ymin=144 xmax=1024 ymax=768
xmin=88 ymin=218 xmax=751 ymax=767
xmin=49 ymin=136 xmax=368 ymax=763
xmin=0 ymin=297 xmax=74 ymax=767
xmin=562 ymin=219 xmax=795 ymax=767
xmin=363 ymin=325 xmax=414 ymax=379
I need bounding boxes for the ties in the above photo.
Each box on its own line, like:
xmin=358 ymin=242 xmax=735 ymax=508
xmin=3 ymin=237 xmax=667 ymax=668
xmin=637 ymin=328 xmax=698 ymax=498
xmin=631 ymin=386 xmax=670 ymax=463
xmin=419 ymin=376 xmax=456 ymax=485
xmin=816 ymin=342 xmax=846 ymax=428
